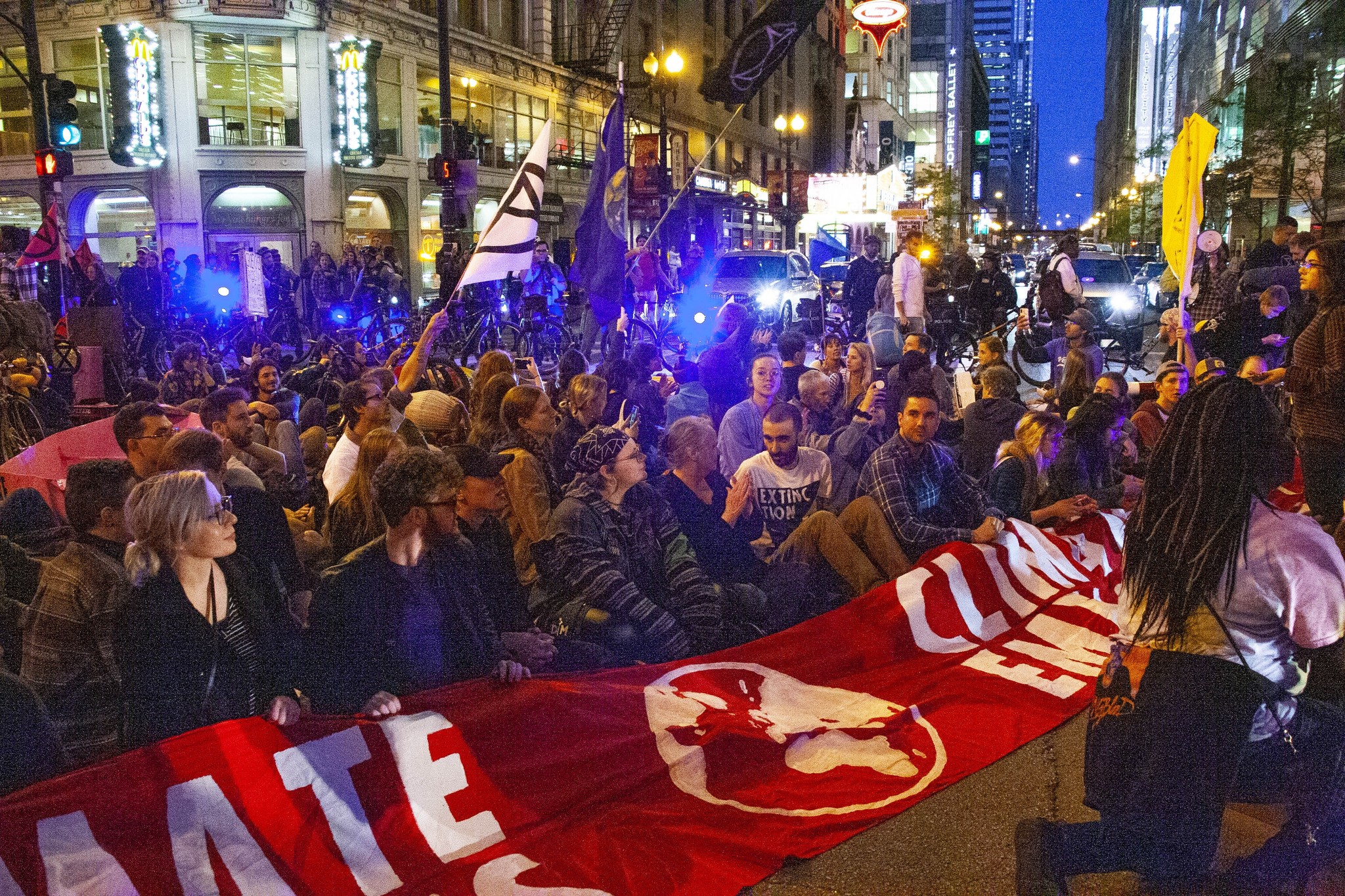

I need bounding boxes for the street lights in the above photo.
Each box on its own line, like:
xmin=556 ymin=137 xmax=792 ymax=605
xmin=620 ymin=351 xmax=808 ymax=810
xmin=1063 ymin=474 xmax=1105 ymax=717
xmin=972 ymin=215 xmax=979 ymax=234
xmin=774 ymin=112 xmax=806 ymax=249
xmin=1066 ymin=214 xmax=1080 ymax=231
xmin=1272 ymin=33 xmax=1324 ymax=223
xmin=1070 ymin=157 xmax=1119 ymax=254
xmin=642 ymin=48 xmax=686 ymax=304
xmin=948 ymin=157 xmax=986 ymax=251
xmin=1076 ymin=192 xmax=1102 ymax=244
xmin=1080 ymin=217 xmax=1101 ymax=243
xmin=1096 ymin=212 xmax=1106 ymax=244
xmin=1121 ymin=187 xmax=1137 ymax=254
xmin=989 ymin=222 xmax=1002 ymax=234
xmin=982 ymin=218 xmax=992 ymax=243
xmin=1135 ymin=174 xmax=1156 ymax=255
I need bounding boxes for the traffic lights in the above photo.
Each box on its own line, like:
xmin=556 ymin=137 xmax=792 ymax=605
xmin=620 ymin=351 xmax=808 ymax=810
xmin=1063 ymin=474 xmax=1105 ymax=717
xmin=40 ymin=152 xmax=56 ymax=174
xmin=440 ymin=159 xmax=451 ymax=179
xmin=49 ymin=77 xmax=81 ymax=148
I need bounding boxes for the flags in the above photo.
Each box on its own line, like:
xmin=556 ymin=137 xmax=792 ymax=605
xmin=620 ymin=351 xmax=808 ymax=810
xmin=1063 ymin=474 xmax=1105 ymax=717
xmin=15 ymin=203 xmax=60 ymax=267
xmin=562 ymin=96 xmax=636 ymax=321
xmin=1162 ymin=113 xmax=1218 ymax=291
xmin=699 ymin=1 xmax=818 ymax=106
xmin=456 ymin=119 xmax=548 ymax=296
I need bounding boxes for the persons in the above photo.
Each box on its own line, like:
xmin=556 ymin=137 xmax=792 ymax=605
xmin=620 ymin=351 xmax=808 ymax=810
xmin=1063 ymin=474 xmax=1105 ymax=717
xmin=0 ymin=226 xmax=1345 ymax=784
xmin=1016 ymin=369 xmax=1345 ymax=896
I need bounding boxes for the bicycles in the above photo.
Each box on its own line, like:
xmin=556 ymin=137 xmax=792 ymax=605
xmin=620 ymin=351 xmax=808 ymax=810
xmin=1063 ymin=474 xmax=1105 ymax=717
xmin=0 ymin=282 xmax=1306 ymax=463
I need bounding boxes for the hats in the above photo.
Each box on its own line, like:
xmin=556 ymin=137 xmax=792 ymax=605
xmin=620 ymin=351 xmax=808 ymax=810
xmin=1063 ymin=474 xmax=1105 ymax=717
xmin=442 ymin=444 xmax=515 ymax=476
xmin=1155 ymin=359 xmax=1189 ymax=380
xmin=1062 ymin=307 xmax=1097 ymax=331
xmin=1159 ymin=308 xmax=1194 ymax=330
xmin=1194 ymin=357 xmax=1237 ymax=377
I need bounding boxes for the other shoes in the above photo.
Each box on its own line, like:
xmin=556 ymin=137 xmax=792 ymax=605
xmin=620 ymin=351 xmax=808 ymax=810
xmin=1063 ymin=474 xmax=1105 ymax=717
xmin=1211 ymin=855 xmax=1277 ymax=896
xmin=1013 ymin=822 xmax=1108 ymax=895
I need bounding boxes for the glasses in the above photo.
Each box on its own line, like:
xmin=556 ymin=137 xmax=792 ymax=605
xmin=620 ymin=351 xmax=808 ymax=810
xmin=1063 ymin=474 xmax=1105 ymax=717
xmin=609 ymin=446 xmax=643 ymax=466
xmin=1066 ymin=319 xmax=1080 ymax=324
xmin=351 ymin=391 xmax=383 ymax=409
xmin=1299 ymin=261 xmax=1328 ymax=273
xmin=126 ymin=427 xmax=176 ymax=441
xmin=1157 ymin=321 xmax=1167 ymax=328
xmin=403 ymin=494 xmax=457 ymax=516
xmin=183 ymin=496 xmax=233 ymax=526
xmin=753 ymin=370 xmax=782 ymax=378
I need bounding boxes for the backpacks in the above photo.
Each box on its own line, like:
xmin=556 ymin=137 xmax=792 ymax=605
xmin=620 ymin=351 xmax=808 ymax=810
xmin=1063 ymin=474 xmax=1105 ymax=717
xmin=1039 ymin=258 xmax=1078 ymax=319
xmin=865 ymin=311 xmax=905 ymax=367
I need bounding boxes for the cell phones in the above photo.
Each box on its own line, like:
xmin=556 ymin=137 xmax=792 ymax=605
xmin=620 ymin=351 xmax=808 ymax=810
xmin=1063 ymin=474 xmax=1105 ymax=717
xmin=1278 ymin=335 xmax=1290 ymax=341
xmin=871 ymin=367 xmax=888 ymax=403
xmin=1019 ymin=308 xmax=1029 ymax=329
xmin=514 ymin=358 xmax=531 ymax=371
xmin=1073 ymin=496 xmax=1090 ymax=508
xmin=1245 ymin=375 xmax=1264 ymax=381
xmin=630 ymin=405 xmax=639 ymax=427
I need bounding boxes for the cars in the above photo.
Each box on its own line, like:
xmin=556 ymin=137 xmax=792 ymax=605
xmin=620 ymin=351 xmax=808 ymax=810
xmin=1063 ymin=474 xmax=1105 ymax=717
xmin=809 ymin=242 xmax=1176 ymax=353
xmin=695 ymin=250 xmax=823 ymax=335
